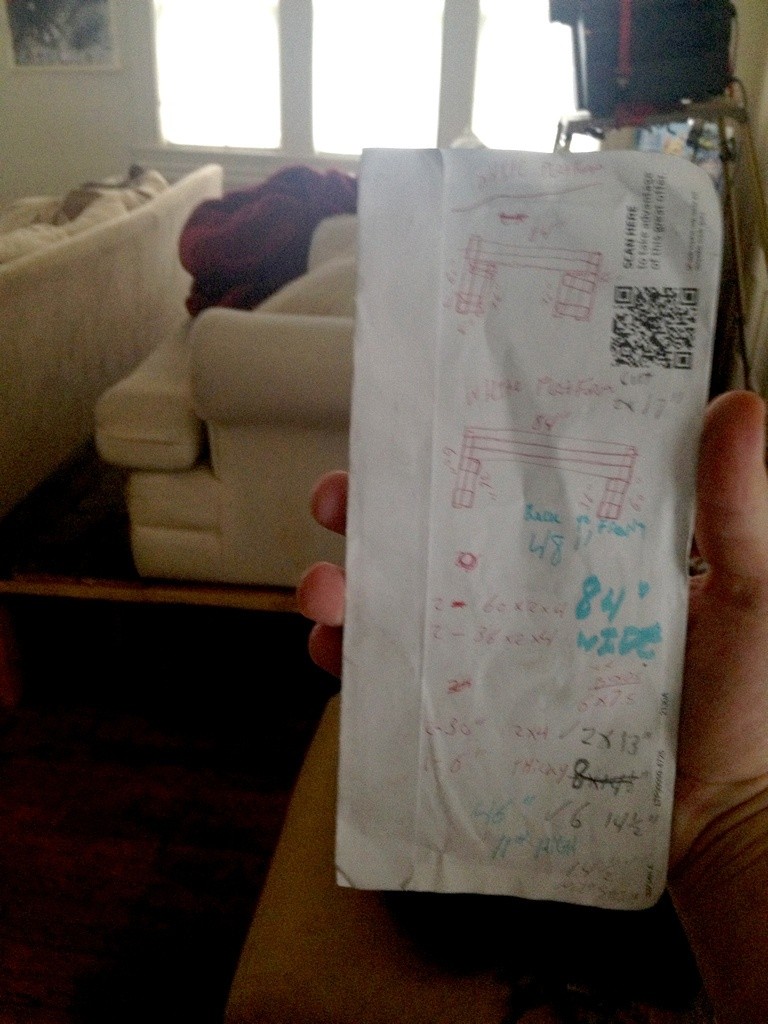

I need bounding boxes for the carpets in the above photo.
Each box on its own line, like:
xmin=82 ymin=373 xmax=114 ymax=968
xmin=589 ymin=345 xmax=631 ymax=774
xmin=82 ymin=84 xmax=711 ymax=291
xmin=0 ymin=440 xmax=340 ymax=1024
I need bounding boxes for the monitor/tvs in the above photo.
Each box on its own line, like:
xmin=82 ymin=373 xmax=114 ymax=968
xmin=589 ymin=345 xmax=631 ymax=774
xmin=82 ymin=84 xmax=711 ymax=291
xmin=573 ymin=0 xmax=731 ymax=116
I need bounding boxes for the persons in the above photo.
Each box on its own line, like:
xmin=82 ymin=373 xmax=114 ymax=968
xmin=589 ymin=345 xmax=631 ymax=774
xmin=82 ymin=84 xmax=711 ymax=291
xmin=296 ymin=389 xmax=768 ymax=1024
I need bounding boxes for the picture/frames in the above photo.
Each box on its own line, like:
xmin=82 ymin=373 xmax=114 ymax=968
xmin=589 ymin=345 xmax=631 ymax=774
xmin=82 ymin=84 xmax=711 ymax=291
xmin=0 ymin=0 xmax=124 ymax=74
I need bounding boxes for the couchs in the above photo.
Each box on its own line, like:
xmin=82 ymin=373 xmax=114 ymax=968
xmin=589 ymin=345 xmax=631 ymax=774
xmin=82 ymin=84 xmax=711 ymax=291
xmin=0 ymin=164 xmax=226 ymax=515
xmin=95 ymin=214 xmax=359 ymax=588
